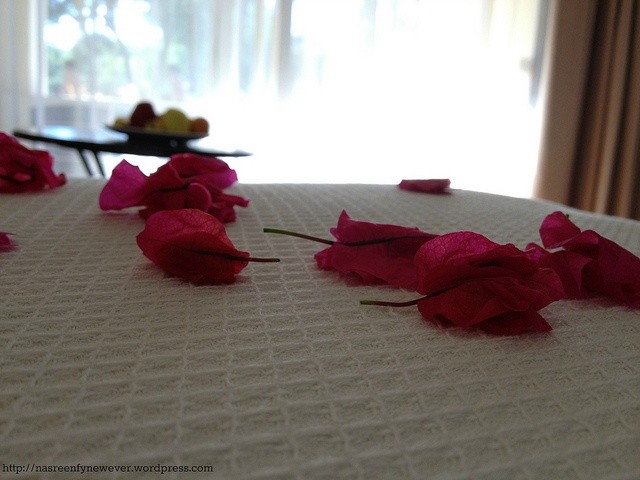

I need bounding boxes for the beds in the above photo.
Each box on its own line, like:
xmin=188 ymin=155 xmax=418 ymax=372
xmin=0 ymin=179 xmax=639 ymax=479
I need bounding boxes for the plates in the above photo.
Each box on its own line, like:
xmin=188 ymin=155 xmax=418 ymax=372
xmin=103 ymin=124 xmax=210 ymax=149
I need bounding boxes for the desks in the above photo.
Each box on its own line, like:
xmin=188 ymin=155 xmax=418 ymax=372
xmin=13 ymin=126 xmax=253 ymax=178
